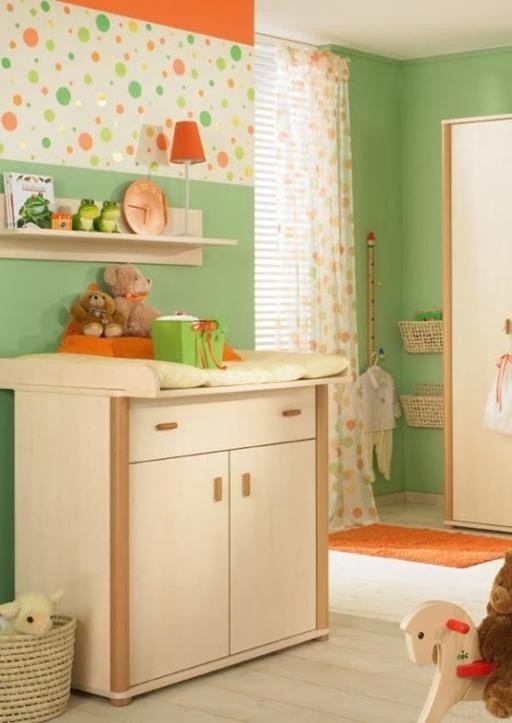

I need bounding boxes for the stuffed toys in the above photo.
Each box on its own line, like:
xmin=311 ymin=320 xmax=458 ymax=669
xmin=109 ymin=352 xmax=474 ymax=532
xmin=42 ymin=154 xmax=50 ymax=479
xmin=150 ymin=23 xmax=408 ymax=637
xmin=477 ymin=550 xmax=512 ymax=720
xmin=103 ymin=263 xmax=162 ymax=338
xmin=70 ymin=292 xmax=125 ymax=337
xmin=0 ymin=589 xmax=64 ymax=636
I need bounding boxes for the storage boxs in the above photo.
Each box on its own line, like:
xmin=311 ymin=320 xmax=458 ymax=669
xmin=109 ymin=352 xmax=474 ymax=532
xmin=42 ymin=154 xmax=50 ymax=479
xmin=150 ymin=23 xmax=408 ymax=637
xmin=152 ymin=320 xmax=225 ymax=369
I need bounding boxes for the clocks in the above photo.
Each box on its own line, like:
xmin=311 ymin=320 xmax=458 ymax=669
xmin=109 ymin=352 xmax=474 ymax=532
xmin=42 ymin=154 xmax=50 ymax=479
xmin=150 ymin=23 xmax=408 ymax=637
xmin=122 ymin=180 xmax=169 ymax=237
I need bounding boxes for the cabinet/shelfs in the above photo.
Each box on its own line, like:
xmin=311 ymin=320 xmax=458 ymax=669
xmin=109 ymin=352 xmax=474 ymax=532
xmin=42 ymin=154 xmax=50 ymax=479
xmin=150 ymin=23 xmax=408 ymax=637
xmin=1 ymin=374 xmax=353 ymax=706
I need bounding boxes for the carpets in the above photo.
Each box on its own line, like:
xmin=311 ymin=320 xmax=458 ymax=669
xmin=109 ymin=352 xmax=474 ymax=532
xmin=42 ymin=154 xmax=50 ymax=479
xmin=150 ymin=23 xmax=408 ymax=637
xmin=328 ymin=523 xmax=511 ymax=568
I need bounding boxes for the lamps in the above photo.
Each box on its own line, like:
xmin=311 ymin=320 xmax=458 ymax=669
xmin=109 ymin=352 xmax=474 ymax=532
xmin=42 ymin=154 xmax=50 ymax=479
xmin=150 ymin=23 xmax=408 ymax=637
xmin=170 ymin=121 xmax=206 ymax=238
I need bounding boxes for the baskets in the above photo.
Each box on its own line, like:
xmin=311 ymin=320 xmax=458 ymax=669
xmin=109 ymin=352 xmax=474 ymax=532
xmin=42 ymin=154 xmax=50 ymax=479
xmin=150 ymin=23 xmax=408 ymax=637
xmin=395 ymin=307 xmax=445 ymax=354
xmin=0 ymin=613 xmax=80 ymax=722
xmin=398 ymin=383 xmax=445 ymax=430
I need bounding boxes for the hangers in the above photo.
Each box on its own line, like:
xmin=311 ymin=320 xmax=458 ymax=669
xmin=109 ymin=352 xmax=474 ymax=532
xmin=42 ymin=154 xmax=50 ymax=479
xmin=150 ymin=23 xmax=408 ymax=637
xmin=370 ymin=351 xmax=380 ymax=372
